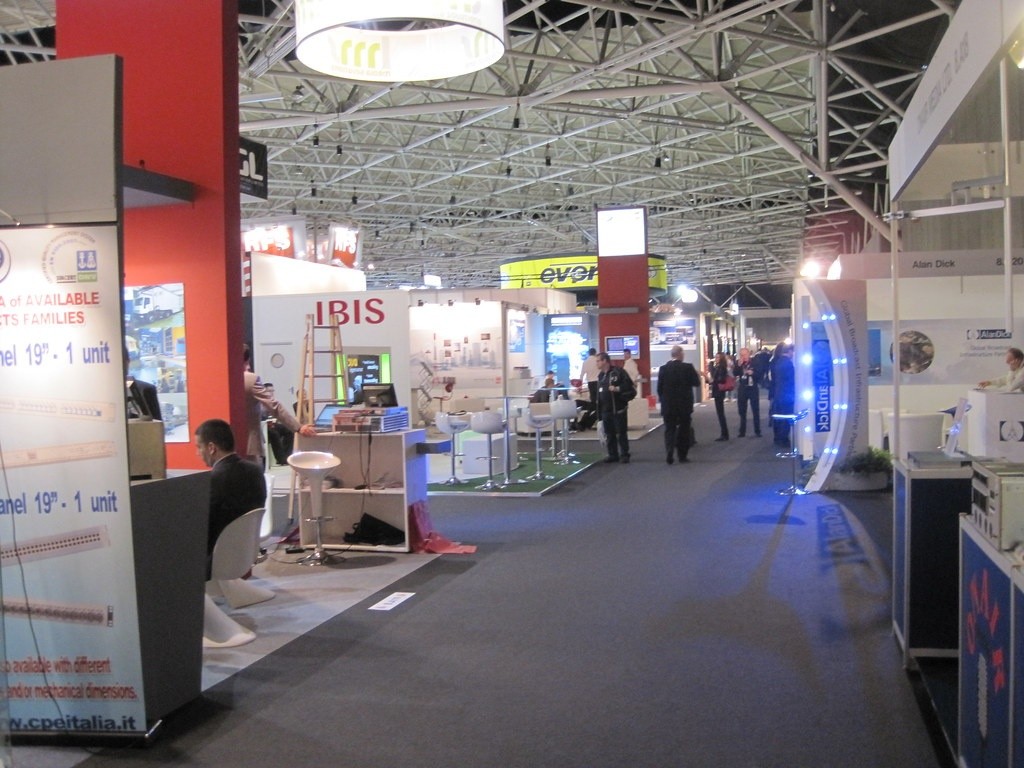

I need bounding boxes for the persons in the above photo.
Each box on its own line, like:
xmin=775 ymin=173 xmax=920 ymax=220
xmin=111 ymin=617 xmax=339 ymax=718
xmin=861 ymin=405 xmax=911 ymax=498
xmin=195 ymin=419 xmax=267 ymax=582
xmin=243 ymin=343 xmax=317 ymax=467
xmin=622 ymin=348 xmax=639 ymax=382
xmin=976 ymin=348 xmax=1024 ymax=394
xmin=706 ymin=341 xmax=786 ymax=427
xmin=596 ymin=352 xmax=637 ymax=464
xmin=774 ymin=344 xmax=794 ymax=449
xmin=657 ymin=345 xmax=700 ymax=466
xmin=708 ymin=351 xmax=729 ymax=442
xmin=580 ymin=347 xmax=601 ymax=403
xmin=124 ymin=345 xmax=162 ymax=422
xmin=732 ymin=348 xmax=763 ymax=438
xmin=528 ymin=370 xmax=570 ymax=408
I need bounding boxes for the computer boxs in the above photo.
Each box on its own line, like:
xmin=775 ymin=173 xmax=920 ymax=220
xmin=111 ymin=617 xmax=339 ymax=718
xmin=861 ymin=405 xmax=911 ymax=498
xmin=333 ymin=413 xmax=409 ymax=432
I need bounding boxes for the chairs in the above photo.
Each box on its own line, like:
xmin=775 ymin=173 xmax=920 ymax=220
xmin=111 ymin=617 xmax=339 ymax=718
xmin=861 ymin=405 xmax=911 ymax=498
xmin=200 ymin=508 xmax=267 ymax=648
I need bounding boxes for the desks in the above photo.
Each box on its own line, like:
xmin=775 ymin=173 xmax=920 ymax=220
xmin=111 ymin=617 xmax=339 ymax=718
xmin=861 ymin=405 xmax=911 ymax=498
xmin=529 ymin=387 xmax=587 ymax=462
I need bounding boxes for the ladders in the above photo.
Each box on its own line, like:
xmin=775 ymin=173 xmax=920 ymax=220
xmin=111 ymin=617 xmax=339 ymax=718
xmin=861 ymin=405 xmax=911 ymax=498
xmin=286 ymin=312 xmax=350 ymax=525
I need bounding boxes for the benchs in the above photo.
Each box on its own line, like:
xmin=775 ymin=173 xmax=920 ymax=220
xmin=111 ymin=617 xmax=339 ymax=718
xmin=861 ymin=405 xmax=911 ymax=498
xmin=867 ymin=408 xmax=944 ymax=454
xmin=517 ymin=397 xmax=649 ymax=438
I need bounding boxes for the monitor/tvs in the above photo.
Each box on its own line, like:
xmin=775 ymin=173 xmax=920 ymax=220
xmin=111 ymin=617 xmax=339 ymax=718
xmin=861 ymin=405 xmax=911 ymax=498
xmin=604 ymin=336 xmax=640 ymax=360
xmin=362 ymin=382 xmax=398 ymax=408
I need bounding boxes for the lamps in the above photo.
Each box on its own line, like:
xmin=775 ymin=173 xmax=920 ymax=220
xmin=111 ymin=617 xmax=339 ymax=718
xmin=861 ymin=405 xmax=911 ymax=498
xmin=294 ymin=0 xmax=506 ymax=83
xmin=291 ymin=85 xmax=663 ymax=239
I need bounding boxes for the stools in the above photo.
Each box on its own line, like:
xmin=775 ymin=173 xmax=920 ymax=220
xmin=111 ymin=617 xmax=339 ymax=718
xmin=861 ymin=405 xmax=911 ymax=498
xmin=772 ymin=407 xmax=811 ymax=496
xmin=939 ymin=404 xmax=971 ymax=454
xmin=286 ymin=450 xmax=347 ymax=567
xmin=436 ymin=398 xmax=581 ymax=491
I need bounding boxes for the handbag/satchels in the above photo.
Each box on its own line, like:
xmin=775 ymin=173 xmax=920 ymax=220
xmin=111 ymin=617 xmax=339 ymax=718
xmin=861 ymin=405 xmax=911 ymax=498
xmin=614 ymin=369 xmax=637 ymax=403
xmin=714 ymin=366 xmax=735 ymax=391
xmin=352 ymin=513 xmax=405 ymax=545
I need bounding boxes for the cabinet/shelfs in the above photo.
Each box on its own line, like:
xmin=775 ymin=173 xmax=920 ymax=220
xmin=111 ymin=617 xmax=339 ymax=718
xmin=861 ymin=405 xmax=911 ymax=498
xmin=298 ymin=428 xmax=426 ymax=553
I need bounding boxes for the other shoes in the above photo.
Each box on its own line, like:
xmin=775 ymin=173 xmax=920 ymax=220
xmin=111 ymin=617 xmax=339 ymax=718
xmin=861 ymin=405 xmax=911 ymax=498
xmin=621 ymin=453 xmax=630 ymax=463
xmin=604 ymin=455 xmax=618 ymax=462
xmin=666 ymin=451 xmax=674 ymax=465
xmin=716 ymin=435 xmax=728 ymax=441
xmin=679 ymin=456 xmax=690 ymax=463
xmin=756 ymin=431 xmax=763 ymax=438
xmin=738 ymin=432 xmax=744 ymax=437
